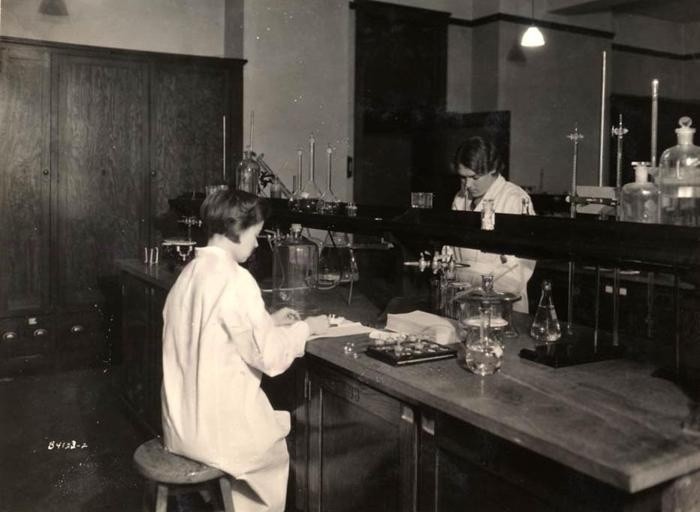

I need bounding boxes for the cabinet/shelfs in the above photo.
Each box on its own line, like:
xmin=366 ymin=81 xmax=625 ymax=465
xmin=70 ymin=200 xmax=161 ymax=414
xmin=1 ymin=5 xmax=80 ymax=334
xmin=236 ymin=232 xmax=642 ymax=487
xmin=0 ymin=35 xmax=247 ymax=376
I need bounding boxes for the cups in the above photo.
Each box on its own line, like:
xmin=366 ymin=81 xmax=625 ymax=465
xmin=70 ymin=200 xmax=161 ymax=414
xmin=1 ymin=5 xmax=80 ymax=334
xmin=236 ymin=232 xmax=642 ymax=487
xmin=411 ymin=193 xmax=433 ymax=209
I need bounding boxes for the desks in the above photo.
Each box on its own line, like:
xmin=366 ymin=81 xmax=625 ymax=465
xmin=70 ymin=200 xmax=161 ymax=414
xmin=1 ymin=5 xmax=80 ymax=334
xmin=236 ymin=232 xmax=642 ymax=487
xmin=114 ymin=256 xmax=699 ymax=512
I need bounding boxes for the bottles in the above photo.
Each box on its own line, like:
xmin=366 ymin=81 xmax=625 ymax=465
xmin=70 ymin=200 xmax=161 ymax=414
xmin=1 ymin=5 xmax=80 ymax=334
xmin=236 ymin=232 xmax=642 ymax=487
xmin=346 ymin=201 xmax=358 ymax=216
xmin=238 ymin=132 xmax=340 ymax=217
xmin=431 ymin=269 xmax=562 ymax=376
xmin=621 ymin=116 xmax=699 ymax=228
xmin=273 ymin=223 xmax=359 ymax=317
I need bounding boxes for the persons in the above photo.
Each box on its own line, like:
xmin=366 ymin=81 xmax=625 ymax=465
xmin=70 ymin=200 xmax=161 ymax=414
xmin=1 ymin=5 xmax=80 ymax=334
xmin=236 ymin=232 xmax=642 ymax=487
xmin=160 ymin=190 xmax=329 ymax=512
xmin=440 ymin=135 xmax=541 ymax=315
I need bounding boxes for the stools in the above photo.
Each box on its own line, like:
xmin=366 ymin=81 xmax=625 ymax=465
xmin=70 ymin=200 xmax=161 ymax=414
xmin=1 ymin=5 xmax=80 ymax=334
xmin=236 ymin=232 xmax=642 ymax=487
xmin=132 ymin=432 xmax=237 ymax=512
